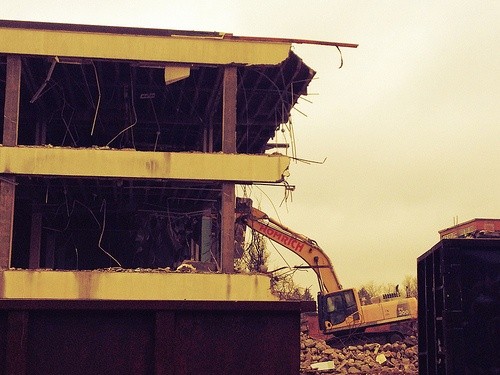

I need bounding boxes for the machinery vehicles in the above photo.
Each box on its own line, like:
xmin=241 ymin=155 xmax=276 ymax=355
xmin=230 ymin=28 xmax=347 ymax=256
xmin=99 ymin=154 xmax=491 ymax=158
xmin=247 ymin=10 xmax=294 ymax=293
xmin=234 ymin=196 xmax=419 ymax=349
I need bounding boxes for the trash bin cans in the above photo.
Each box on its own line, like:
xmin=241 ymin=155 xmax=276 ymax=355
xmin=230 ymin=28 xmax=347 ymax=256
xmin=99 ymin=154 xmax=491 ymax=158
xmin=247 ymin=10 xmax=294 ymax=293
xmin=415 ymin=235 xmax=499 ymax=375
